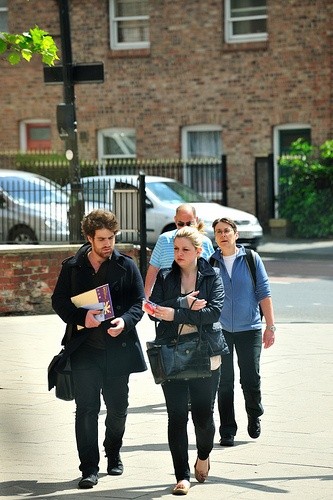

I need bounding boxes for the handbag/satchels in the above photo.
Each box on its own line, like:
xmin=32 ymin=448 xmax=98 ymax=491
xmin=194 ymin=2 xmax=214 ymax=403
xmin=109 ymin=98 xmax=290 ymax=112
xmin=47 ymin=348 xmax=75 ymax=401
xmin=145 ymin=298 xmax=213 ymax=385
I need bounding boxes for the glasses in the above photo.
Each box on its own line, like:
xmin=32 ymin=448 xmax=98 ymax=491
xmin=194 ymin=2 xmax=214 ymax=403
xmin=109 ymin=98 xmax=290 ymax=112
xmin=176 ymin=220 xmax=193 ymax=226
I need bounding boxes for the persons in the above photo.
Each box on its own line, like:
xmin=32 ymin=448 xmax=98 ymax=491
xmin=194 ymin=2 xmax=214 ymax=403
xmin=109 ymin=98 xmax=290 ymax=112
xmin=144 ymin=204 xmax=215 ymax=300
xmin=50 ymin=209 xmax=146 ymax=486
xmin=207 ymin=218 xmax=276 ymax=446
xmin=148 ymin=226 xmax=225 ymax=495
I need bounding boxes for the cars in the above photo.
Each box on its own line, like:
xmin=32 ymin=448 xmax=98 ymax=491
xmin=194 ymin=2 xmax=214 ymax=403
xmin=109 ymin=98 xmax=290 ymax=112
xmin=61 ymin=174 xmax=263 ymax=254
xmin=0 ymin=169 xmax=94 ymax=244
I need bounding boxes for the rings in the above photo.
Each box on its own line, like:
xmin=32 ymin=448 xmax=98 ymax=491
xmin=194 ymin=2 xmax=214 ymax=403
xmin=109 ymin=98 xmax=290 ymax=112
xmin=161 ymin=315 xmax=163 ymax=316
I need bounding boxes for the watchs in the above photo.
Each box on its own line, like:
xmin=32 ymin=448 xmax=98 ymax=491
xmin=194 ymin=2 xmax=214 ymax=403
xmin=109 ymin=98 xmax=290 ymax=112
xmin=266 ymin=325 xmax=276 ymax=332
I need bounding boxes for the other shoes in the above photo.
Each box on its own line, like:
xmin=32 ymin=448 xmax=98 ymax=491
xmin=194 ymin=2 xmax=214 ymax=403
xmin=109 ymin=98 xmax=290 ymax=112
xmin=172 ymin=483 xmax=189 ymax=495
xmin=220 ymin=434 xmax=234 ymax=446
xmin=248 ymin=414 xmax=261 ymax=439
xmin=104 ymin=449 xmax=124 ymax=475
xmin=194 ymin=455 xmax=211 ymax=483
xmin=78 ymin=470 xmax=99 ymax=489
